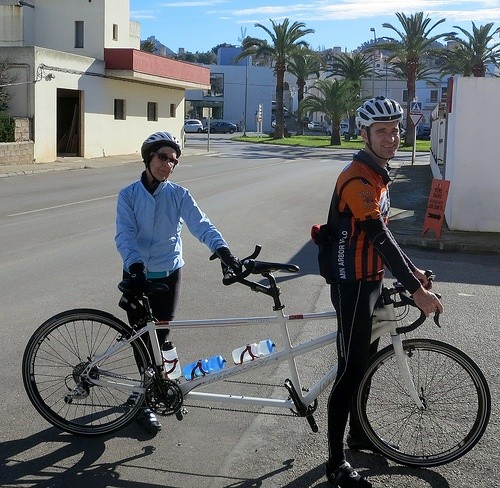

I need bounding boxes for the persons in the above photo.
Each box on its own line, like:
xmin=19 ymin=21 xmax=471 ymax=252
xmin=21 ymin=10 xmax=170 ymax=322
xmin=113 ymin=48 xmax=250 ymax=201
xmin=114 ymin=131 xmax=244 ymax=431
xmin=320 ymin=96 xmax=443 ymax=488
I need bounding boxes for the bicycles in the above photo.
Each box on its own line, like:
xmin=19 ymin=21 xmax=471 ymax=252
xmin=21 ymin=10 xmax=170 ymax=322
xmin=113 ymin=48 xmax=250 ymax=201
xmin=20 ymin=242 xmax=494 ymax=469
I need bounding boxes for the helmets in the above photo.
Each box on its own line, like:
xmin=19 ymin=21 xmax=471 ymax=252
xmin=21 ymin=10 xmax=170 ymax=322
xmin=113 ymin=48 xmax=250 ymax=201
xmin=356 ymin=95 xmax=403 ymax=132
xmin=142 ymin=132 xmax=181 ymax=159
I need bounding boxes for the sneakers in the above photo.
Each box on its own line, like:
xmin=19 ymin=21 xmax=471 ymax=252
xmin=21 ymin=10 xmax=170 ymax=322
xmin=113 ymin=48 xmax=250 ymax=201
xmin=347 ymin=428 xmax=401 ymax=452
xmin=324 ymin=458 xmax=374 ymax=488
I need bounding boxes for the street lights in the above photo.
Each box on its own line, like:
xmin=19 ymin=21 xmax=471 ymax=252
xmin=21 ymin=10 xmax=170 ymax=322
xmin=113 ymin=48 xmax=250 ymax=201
xmin=370 ymin=27 xmax=377 ymax=97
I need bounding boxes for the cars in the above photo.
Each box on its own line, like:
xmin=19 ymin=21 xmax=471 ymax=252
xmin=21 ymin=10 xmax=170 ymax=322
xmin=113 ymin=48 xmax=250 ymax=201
xmin=308 ymin=120 xmax=327 ymax=132
xmin=203 ymin=119 xmax=237 ymax=134
xmin=184 ymin=118 xmax=204 ymax=133
xmin=325 ymin=122 xmax=350 ymax=137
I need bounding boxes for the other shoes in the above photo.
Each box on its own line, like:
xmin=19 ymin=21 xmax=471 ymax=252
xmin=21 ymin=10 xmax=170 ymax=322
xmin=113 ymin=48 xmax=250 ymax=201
xmin=137 ymin=406 xmax=163 ymax=432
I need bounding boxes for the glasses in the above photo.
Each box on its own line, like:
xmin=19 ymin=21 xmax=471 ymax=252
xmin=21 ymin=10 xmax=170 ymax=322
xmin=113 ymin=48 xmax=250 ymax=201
xmin=149 ymin=151 xmax=179 ymax=166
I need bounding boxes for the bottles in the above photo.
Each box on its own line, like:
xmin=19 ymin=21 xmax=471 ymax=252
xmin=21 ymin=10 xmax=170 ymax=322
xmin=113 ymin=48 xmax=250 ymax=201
xmin=182 ymin=354 xmax=227 ymax=381
xmin=161 ymin=340 xmax=182 ymax=379
xmin=232 ymin=339 xmax=275 ymax=364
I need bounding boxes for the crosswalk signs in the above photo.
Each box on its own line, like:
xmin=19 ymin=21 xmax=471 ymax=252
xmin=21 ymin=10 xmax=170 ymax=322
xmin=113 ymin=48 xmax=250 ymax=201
xmin=410 ymin=102 xmax=423 ymax=113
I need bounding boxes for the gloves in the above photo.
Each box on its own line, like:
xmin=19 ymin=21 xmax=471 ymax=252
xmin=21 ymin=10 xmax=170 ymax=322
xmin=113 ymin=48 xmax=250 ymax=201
xmin=128 ymin=263 xmax=152 ymax=297
xmin=210 ymin=248 xmax=241 ymax=276
